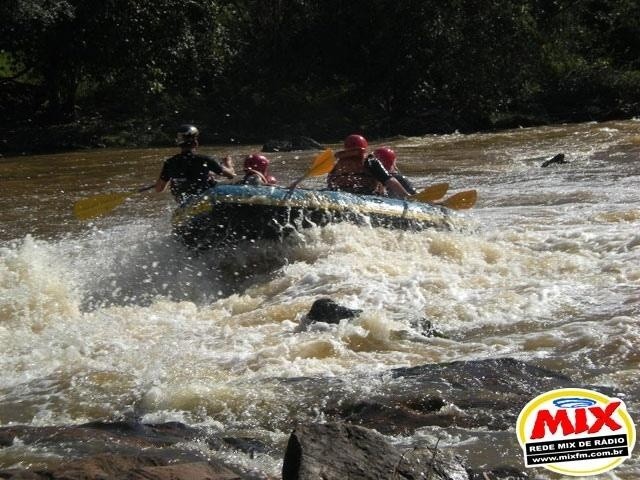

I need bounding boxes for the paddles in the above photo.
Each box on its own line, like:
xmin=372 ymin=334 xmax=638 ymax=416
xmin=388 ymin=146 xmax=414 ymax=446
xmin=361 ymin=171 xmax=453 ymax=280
xmin=75 ymin=181 xmax=158 ymax=221
xmin=435 ymin=191 xmax=478 ymax=209
xmin=404 ymin=184 xmax=449 ymax=203
xmin=290 ymin=148 xmax=336 ymax=188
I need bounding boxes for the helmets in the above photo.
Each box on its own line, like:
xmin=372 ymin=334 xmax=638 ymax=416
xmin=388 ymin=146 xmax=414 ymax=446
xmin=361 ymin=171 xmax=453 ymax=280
xmin=174 ymin=124 xmax=199 ymax=147
xmin=373 ymin=147 xmax=396 ymax=172
xmin=345 ymin=134 xmax=368 ymax=159
xmin=243 ymin=153 xmax=278 ymax=186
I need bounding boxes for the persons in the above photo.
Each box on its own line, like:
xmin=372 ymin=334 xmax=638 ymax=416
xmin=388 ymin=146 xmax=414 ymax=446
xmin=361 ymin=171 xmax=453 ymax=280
xmin=154 ymin=123 xmax=236 ymax=205
xmin=237 ymin=153 xmax=297 ymax=190
xmin=370 ymin=147 xmax=442 ymax=205
xmin=326 ymin=134 xmax=414 ymax=199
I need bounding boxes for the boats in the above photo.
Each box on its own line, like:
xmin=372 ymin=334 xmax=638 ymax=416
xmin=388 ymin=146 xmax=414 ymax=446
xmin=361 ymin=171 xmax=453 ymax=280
xmin=165 ymin=183 xmax=456 ymax=260
